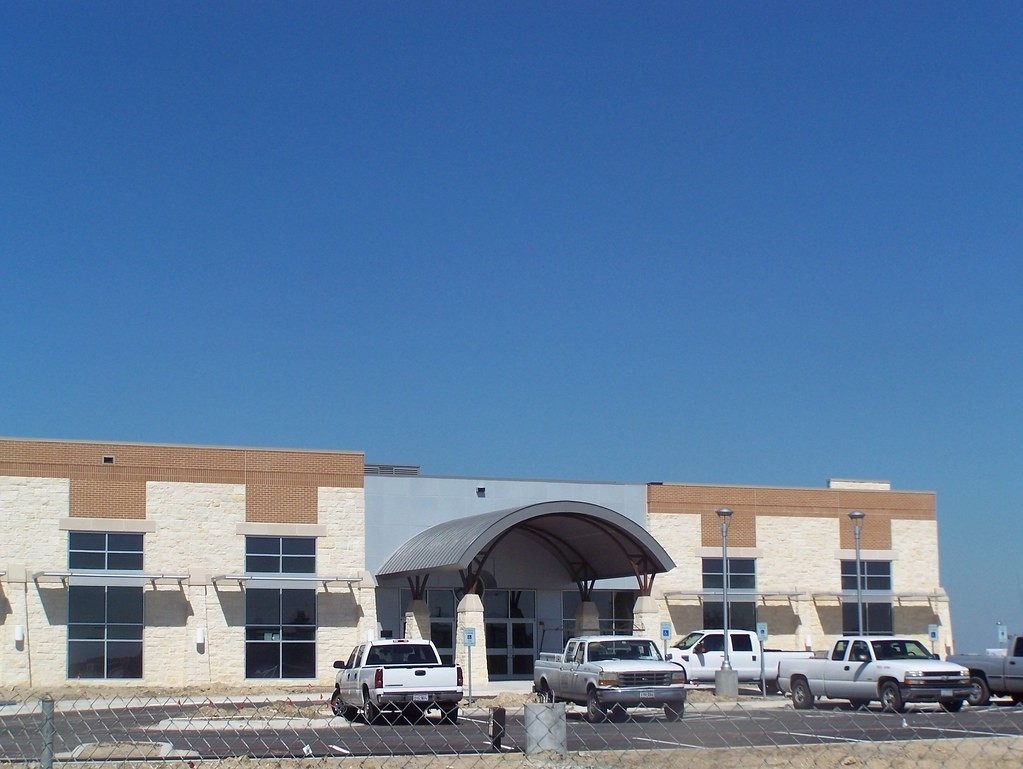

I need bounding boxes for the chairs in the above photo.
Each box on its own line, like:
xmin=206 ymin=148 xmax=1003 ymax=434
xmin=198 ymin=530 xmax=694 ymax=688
xmin=616 ymin=649 xmax=628 ymax=659
xmin=407 ymin=654 xmax=419 ymax=662
xmin=368 ymin=654 xmax=379 ymax=665
xmin=582 ymin=650 xmax=600 ymax=662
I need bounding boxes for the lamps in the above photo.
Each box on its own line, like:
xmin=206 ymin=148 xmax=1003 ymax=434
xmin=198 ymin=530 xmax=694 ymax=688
xmin=805 ymin=636 xmax=811 ymax=647
xmin=476 ymin=488 xmax=486 ymax=492
xmin=15 ymin=625 xmax=24 ymax=641
xmin=197 ymin=628 xmax=205 ymax=644
xmin=102 ymin=455 xmax=115 ymax=464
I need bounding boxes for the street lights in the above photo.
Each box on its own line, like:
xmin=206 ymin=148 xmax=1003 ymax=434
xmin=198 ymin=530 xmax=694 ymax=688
xmin=848 ymin=510 xmax=865 ymax=650
xmin=716 ymin=506 xmax=735 ymax=670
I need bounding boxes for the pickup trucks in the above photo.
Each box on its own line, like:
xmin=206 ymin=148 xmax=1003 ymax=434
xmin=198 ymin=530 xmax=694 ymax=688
xmin=946 ymin=634 xmax=1023 ymax=705
xmin=667 ymin=629 xmax=815 ymax=694
xmin=532 ymin=635 xmax=686 ymax=724
xmin=777 ymin=635 xmax=971 ymax=713
xmin=331 ymin=639 xmax=464 ymax=723
